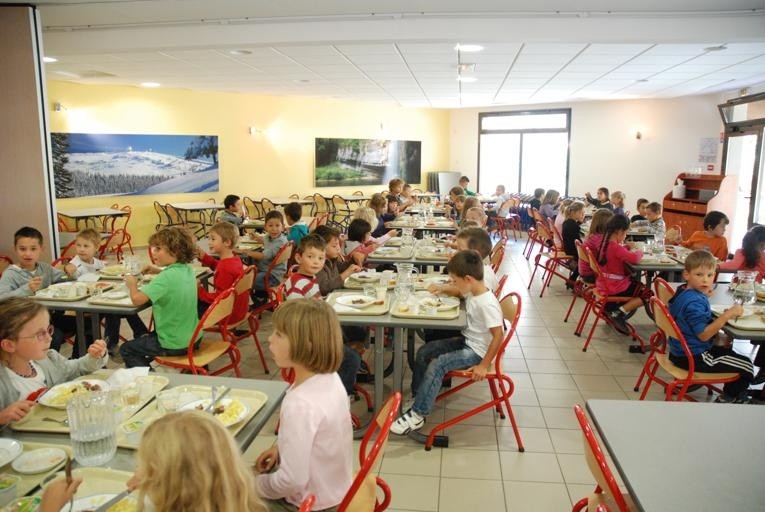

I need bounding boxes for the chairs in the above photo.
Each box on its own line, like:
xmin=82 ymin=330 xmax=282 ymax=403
xmin=420 ymin=292 xmax=529 ymax=452
xmin=334 ymin=390 xmax=401 ymax=511
xmin=295 ymin=493 xmax=317 ymax=511
xmin=571 ymin=403 xmax=642 ymax=511
xmin=272 ymin=281 xmax=373 ymax=436
xmin=0 ymin=186 xmax=765 ymax=402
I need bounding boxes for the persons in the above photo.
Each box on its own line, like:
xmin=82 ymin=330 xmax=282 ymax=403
xmin=530 ymin=186 xmax=765 ymax=404
xmin=0 ymin=173 xmax=509 ymax=512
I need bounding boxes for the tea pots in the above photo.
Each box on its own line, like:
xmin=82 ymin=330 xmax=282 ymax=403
xmin=733 ymin=267 xmax=760 ymax=306
xmin=392 ymin=261 xmax=419 ymax=295
xmin=398 ymin=228 xmax=415 ymax=248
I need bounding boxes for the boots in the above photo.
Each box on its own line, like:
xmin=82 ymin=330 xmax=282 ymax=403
xmin=608 ymin=308 xmax=629 ymax=334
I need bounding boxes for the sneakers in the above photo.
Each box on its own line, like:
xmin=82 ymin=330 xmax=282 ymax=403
xmin=390 ymin=409 xmax=424 ymax=435
xmin=716 ymin=396 xmax=760 ymax=404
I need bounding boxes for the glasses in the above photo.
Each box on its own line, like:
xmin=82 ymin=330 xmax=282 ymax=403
xmin=18 ymin=325 xmax=54 ymax=340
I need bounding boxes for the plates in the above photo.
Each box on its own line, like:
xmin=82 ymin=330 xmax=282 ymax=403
xmin=11 ymin=445 xmax=67 ymax=474
xmin=710 ymin=302 xmax=754 ymax=318
xmin=107 ymin=290 xmax=129 ymax=302
xmin=0 ymin=436 xmax=24 ymax=470
xmin=98 ymin=264 xmax=123 ymax=276
xmin=423 ymin=273 xmax=455 ymax=287
xmin=335 ymin=295 xmax=377 ymax=308
xmin=351 ymin=272 xmax=380 ymax=283
xmin=58 ymin=492 xmax=139 ymax=512
xmin=422 ymin=297 xmax=460 ymax=310
xmin=179 ymin=398 xmax=248 ymax=426
xmin=372 ymin=230 xmax=453 ymax=258
xmin=39 ymin=380 xmax=108 ymax=408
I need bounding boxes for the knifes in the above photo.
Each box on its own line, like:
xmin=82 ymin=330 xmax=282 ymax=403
xmin=95 ymin=487 xmax=136 ymax=511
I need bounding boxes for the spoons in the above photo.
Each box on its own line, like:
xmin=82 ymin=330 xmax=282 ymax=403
xmin=733 ymin=297 xmax=745 ymax=324
xmin=207 ymin=386 xmax=217 ymax=414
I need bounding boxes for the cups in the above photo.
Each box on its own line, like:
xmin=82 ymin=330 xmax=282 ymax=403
xmin=7 ymin=496 xmax=40 ymax=512
xmin=426 ymin=265 xmax=435 ymax=275
xmin=153 ymin=389 xmax=182 ymax=418
xmin=124 ymin=426 xmax=143 ymax=446
xmin=362 ymin=268 xmax=411 ymax=307
xmin=637 ymin=233 xmax=681 ymax=258
xmin=0 ymin=484 xmax=18 ymax=507
xmin=684 ymin=165 xmax=705 ymax=180
xmin=107 ymin=378 xmax=142 ymax=406
xmin=38 ymin=471 xmax=66 ymax=488
xmin=49 ymin=284 xmax=102 ymax=299
xmin=67 ymin=395 xmax=118 ymax=465
xmin=127 ymin=261 xmax=141 ymax=276
xmin=400 ymin=199 xmax=445 ymax=227
